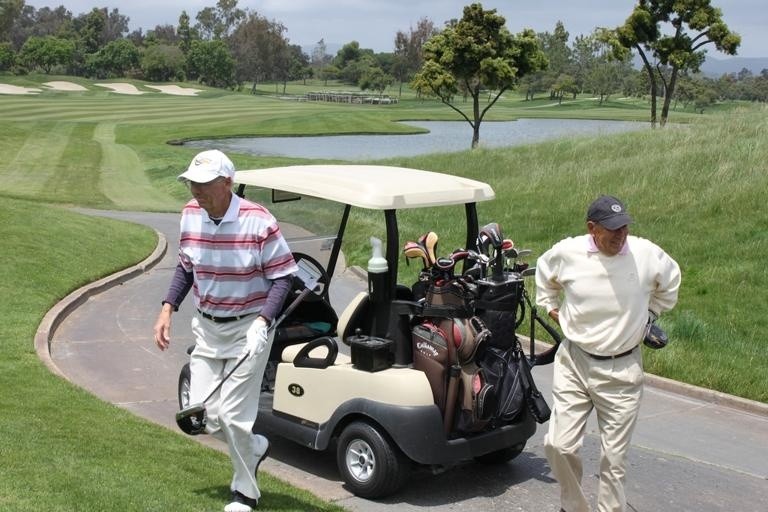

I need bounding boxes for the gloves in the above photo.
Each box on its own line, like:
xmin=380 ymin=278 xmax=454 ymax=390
xmin=241 ymin=319 xmax=270 ymax=360
xmin=642 ymin=309 xmax=660 ymax=343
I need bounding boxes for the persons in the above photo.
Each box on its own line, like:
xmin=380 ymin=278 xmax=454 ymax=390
xmin=531 ymin=193 xmax=684 ymax=509
xmin=149 ymin=146 xmax=300 ymax=512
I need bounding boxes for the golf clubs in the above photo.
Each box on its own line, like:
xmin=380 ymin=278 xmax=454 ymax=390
xmin=403 ymin=231 xmax=469 ymax=287
xmin=175 ymin=288 xmax=313 ymax=436
xmin=463 ymin=223 xmax=536 ymax=282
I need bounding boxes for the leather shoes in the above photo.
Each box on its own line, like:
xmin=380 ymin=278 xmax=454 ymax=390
xmin=251 ymin=432 xmax=271 ymax=477
xmin=224 ymin=489 xmax=257 ymax=512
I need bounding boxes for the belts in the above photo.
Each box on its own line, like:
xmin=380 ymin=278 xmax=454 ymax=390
xmin=590 ymin=344 xmax=639 ymax=360
xmin=196 ymin=306 xmax=253 ymax=323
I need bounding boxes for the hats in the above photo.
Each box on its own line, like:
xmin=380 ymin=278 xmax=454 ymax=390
xmin=175 ymin=149 xmax=237 ymax=184
xmin=586 ymin=194 xmax=637 ymax=231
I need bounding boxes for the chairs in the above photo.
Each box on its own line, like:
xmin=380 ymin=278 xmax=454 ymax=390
xmin=280 ymin=285 xmax=412 ymax=368
